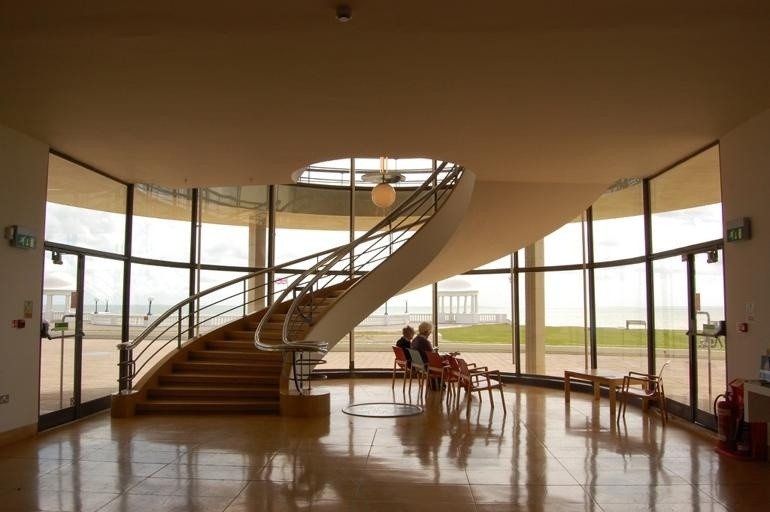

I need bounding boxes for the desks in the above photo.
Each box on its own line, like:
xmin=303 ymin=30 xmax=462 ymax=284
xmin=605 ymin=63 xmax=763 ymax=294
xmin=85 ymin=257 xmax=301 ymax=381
xmin=565 ymin=368 xmax=648 ymax=424
xmin=744 ymin=381 xmax=770 ymax=423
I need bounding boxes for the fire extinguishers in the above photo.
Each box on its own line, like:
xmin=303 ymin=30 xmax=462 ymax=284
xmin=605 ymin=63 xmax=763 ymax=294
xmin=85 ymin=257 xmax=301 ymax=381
xmin=714 ymin=391 xmax=735 ymax=450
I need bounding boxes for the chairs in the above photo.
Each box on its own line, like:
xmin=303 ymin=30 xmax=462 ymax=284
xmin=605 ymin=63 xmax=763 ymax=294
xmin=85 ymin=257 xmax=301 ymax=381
xmin=393 ymin=346 xmax=506 ymax=419
xmin=617 ymin=359 xmax=671 ymax=427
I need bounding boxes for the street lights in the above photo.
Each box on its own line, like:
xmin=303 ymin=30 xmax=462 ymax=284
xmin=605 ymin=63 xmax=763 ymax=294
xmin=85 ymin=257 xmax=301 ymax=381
xmin=147 ymin=297 xmax=154 ymax=314
xmin=94 ymin=298 xmax=110 ymax=314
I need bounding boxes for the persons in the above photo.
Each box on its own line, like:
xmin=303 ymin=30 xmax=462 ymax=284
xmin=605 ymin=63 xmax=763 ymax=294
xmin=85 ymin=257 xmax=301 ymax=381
xmin=411 ymin=321 xmax=451 ymax=371
xmin=396 ymin=325 xmax=446 ymax=390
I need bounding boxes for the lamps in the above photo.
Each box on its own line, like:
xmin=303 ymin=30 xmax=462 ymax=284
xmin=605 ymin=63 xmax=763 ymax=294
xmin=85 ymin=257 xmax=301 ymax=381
xmin=361 ymin=156 xmax=406 ymax=208
